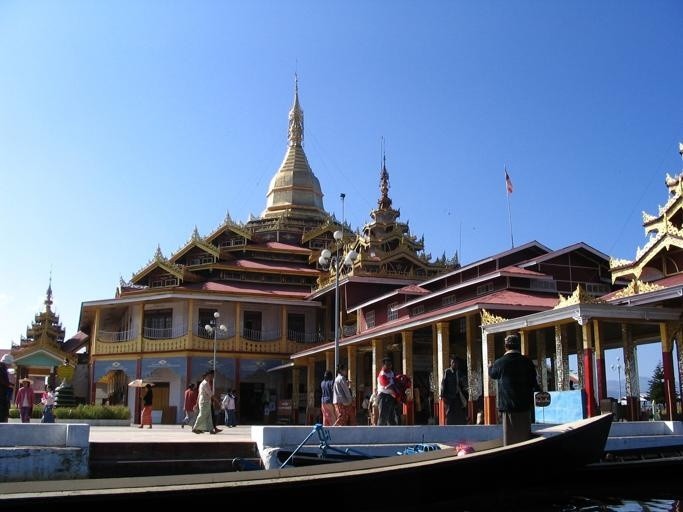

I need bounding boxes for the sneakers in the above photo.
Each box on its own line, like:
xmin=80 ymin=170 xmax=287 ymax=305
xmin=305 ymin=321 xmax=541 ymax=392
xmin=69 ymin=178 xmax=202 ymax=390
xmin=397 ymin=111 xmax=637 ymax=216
xmin=137 ymin=424 xmax=236 ymax=434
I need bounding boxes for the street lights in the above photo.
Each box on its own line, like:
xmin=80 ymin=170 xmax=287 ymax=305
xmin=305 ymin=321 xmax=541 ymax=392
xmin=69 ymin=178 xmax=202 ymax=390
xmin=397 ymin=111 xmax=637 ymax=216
xmin=445 ymin=209 xmax=478 ymax=266
xmin=203 ymin=310 xmax=226 ymax=394
xmin=337 ymin=192 xmax=346 ymax=232
xmin=319 ymin=228 xmax=356 ymax=381
xmin=610 ymin=356 xmax=626 ymax=401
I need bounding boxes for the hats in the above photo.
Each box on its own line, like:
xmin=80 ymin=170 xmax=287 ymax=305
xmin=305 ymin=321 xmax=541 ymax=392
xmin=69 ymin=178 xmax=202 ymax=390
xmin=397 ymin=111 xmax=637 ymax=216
xmin=17 ymin=377 xmax=34 ymax=386
xmin=504 ymin=334 xmax=520 ymax=345
xmin=0 ymin=353 xmax=14 ymax=365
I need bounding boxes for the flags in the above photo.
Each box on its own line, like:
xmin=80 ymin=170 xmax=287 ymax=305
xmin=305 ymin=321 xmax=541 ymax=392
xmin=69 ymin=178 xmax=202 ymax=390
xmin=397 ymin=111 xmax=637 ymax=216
xmin=505 ymin=168 xmax=513 ymax=193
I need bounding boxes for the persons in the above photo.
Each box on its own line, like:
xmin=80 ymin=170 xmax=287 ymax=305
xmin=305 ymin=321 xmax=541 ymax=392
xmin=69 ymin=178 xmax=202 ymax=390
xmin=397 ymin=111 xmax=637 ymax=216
xmin=15 ymin=377 xmax=35 ymax=423
xmin=182 ymin=369 xmax=238 ymax=434
xmin=138 ymin=384 xmax=153 ymax=428
xmin=323 ymin=357 xmax=467 ymax=426
xmin=487 ymin=333 xmax=541 ymax=447
xmin=0 ymin=354 xmax=16 ymax=423
xmin=40 ymin=385 xmax=56 ymax=423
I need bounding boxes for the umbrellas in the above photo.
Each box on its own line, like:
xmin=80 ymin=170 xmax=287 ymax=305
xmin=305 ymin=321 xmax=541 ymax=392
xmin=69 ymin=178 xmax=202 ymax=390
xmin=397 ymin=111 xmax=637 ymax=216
xmin=128 ymin=378 xmax=156 ymax=399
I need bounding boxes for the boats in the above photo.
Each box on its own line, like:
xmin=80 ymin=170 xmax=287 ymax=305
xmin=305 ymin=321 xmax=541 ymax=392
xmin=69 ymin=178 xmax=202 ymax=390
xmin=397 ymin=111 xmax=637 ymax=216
xmin=1 ymin=360 xmax=615 ymax=512
